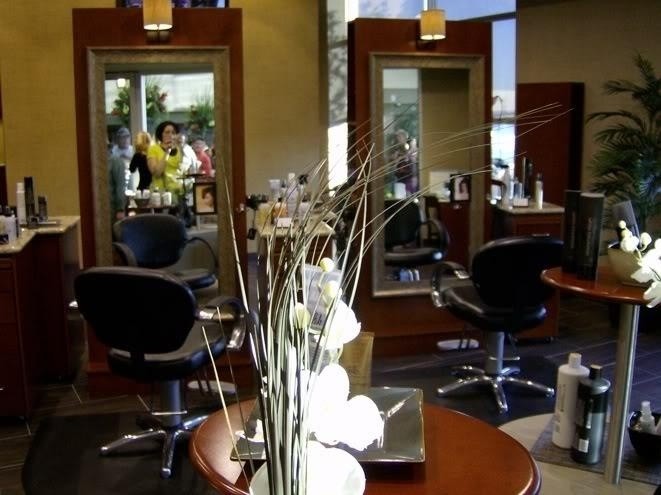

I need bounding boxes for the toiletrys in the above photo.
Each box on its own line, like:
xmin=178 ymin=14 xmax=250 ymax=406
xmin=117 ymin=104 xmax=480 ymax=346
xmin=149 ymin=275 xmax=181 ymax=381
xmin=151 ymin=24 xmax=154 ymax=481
xmin=552 ymin=352 xmax=610 ymax=465
xmin=0 ymin=177 xmax=47 ymax=241
xmin=136 ymin=187 xmax=171 ymax=207
xmin=255 ymin=171 xmax=316 ymax=229
xmin=500 ymin=157 xmax=544 ymax=208
xmin=259 ymin=172 xmax=311 ymax=227
xmin=640 ymin=400 xmax=655 ymax=432
xmin=1 ymin=175 xmax=50 ymax=247
xmin=500 ymin=156 xmax=545 ymax=210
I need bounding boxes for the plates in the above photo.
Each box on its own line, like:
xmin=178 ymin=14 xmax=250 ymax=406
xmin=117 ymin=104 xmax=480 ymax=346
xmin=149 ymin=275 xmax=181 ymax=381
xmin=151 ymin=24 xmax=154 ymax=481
xmin=232 ymin=384 xmax=427 ymax=465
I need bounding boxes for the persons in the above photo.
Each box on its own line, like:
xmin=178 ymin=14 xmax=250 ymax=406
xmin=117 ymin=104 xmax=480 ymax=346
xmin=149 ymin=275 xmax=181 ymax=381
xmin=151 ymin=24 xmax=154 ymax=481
xmin=393 ymin=128 xmax=411 ymax=178
xmin=456 ymin=178 xmax=470 ymax=201
xmin=104 ymin=120 xmax=217 ymax=226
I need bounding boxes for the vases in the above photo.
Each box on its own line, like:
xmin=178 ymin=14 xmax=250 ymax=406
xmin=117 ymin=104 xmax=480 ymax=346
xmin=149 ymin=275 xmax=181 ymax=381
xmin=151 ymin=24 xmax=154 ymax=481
xmin=250 ymin=446 xmax=365 ymax=495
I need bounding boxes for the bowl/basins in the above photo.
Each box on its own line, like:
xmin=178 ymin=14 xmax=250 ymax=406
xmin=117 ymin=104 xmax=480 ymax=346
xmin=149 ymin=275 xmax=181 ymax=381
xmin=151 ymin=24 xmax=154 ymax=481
xmin=133 ymin=198 xmax=149 ymax=208
xmin=606 ymin=241 xmax=654 ymax=290
xmin=628 ymin=410 xmax=661 ymax=461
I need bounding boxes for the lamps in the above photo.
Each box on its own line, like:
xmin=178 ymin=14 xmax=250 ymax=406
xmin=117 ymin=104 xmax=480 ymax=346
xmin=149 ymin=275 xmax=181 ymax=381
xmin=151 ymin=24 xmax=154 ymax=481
xmin=142 ymin=0 xmax=174 ymax=31
xmin=417 ymin=0 xmax=449 ymax=42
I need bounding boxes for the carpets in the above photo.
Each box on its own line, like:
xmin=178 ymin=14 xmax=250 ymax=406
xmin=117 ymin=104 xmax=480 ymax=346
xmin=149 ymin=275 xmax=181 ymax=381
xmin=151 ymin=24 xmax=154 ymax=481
xmin=22 ymin=356 xmax=560 ymax=495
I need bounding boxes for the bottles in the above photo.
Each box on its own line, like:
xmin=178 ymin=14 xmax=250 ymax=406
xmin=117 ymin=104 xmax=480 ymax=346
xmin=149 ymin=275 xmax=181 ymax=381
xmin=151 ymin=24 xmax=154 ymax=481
xmin=0 ymin=174 xmax=48 ymax=246
xmin=554 ymin=351 xmax=613 ymax=466
xmin=150 ymin=188 xmax=173 ymax=207
xmin=562 ymin=188 xmax=606 ymax=280
xmin=500 ymin=155 xmax=545 ymax=210
xmin=611 ymin=200 xmax=643 ymax=250
xmin=259 ymin=173 xmax=311 ymax=233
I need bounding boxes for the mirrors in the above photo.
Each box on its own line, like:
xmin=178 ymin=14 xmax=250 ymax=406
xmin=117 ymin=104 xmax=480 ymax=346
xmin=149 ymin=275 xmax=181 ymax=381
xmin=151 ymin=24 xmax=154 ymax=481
xmin=86 ymin=45 xmax=237 ymax=310
xmin=371 ymin=51 xmax=484 ymax=298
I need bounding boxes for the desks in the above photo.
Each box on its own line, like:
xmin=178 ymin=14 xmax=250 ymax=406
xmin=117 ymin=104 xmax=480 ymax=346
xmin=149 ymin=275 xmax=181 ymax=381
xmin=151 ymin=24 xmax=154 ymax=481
xmin=187 ymin=392 xmax=543 ymax=495
xmin=537 ymin=258 xmax=661 ymax=486
xmin=116 ymin=200 xmax=177 ymax=214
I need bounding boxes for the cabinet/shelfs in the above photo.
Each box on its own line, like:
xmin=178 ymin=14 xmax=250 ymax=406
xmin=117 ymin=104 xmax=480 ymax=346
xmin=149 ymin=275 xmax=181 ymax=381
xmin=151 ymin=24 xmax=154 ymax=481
xmin=255 ymin=217 xmax=338 ymax=304
xmin=0 ymin=231 xmax=41 ymax=416
xmin=18 ymin=215 xmax=84 ymax=379
xmin=492 ymin=197 xmax=565 ymax=342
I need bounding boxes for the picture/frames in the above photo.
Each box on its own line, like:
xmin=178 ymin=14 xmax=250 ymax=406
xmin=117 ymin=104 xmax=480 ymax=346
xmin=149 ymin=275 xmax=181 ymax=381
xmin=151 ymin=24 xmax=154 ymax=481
xmin=193 ymin=182 xmax=217 ymax=216
xmin=451 ymin=174 xmax=473 ymax=203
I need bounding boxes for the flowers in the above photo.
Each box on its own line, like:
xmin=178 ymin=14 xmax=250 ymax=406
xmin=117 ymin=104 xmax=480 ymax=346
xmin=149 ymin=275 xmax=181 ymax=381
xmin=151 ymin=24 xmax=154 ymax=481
xmin=221 ymin=103 xmax=574 ymax=495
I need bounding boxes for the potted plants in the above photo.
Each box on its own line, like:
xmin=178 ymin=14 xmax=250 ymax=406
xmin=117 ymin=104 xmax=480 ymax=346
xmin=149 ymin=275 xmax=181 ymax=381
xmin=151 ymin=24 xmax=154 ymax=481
xmin=581 ymin=51 xmax=661 ymax=333
xmin=112 ymin=82 xmax=165 ymax=132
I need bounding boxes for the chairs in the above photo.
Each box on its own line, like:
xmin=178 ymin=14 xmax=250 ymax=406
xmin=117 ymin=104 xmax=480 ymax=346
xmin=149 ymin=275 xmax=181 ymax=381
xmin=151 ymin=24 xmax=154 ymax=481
xmin=111 ymin=215 xmax=219 ymax=292
xmin=385 ymin=199 xmax=451 ymax=280
xmin=432 ymin=232 xmax=564 ymax=416
xmin=72 ymin=266 xmax=248 ymax=477
